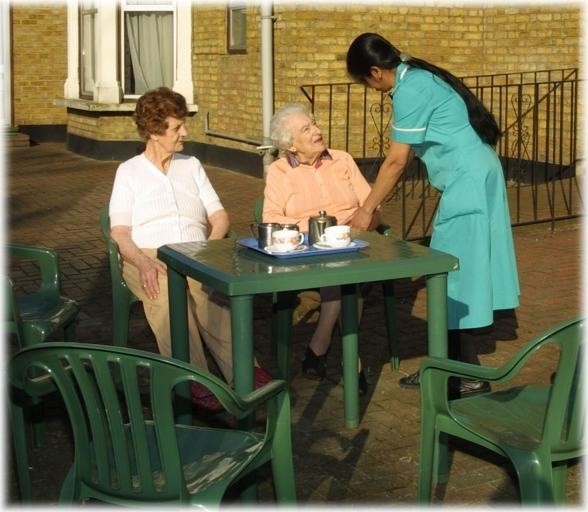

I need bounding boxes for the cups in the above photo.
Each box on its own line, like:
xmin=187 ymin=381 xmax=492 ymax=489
xmin=249 ymin=222 xmax=282 ymax=249
xmin=272 ymin=227 xmax=305 ymax=250
xmin=319 ymin=225 xmax=351 ymax=247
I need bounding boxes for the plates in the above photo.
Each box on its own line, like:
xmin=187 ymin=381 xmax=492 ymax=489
xmin=263 ymin=245 xmax=307 ymax=256
xmin=312 ymin=240 xmax=356 ymax=250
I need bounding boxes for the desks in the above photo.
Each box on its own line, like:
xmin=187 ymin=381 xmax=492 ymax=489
xmin=155 ymin=229 xmax=460 ymax=434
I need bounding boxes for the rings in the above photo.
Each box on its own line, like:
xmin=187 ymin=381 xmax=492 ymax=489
xmin=142 ymin=284 xmax=147 ymax=288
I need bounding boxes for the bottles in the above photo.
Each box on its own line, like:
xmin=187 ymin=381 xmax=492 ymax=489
xmin=308 ymin=210 xmax=338 ymax=246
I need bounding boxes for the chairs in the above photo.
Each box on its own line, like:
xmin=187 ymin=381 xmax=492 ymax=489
xmin=414 ymin=316 xmax=583 ymax=506
xmin=102 ymin=206 xmax=238 ymax=350
xmin=10 ymin=340 xmax=300 ymax=506
xmin=6 ymin=244 xmax=93 ymax=501
xmin=252 ymin=199 xmax=402 ymax=372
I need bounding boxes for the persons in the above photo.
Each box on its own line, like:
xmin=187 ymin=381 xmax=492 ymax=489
xmin=343 ymin=32 xmax=521 ymax=399
xmin=107 ymin=87 xmax=271 ymax=415
xmin=261 ymin=103 xmax=386 ymax=394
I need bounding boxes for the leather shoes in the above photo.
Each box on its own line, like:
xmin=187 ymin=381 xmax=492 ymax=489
xmin=447 ymin=378 xmax=490 ymax=400
xmin=301 ymin=346 xmax=329 ymax=382
xmin=358 ymin=369 xmax=366 ymax=396
xmin=254 ymin=367 xmax=272 ymax=389
xmin=191 ymin=382 xmax=223 ymax=411
xmin=398 ymin=372 xmax=419 ymax=389
